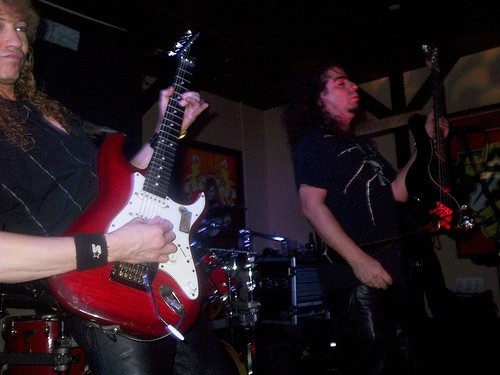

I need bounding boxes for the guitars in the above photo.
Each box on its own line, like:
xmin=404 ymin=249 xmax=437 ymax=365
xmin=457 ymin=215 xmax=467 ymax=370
xmin=47 ymin=30 xmax=206 ymax=344
xmin=405 ymin=43 xmax=475 ymax=232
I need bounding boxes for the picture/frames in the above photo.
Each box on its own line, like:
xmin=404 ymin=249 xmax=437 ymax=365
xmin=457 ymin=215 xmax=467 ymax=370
xmin=183 ymin=140 xmax=245 ymax=207
xmin=446 ymin=102 xmax=500 ymax=260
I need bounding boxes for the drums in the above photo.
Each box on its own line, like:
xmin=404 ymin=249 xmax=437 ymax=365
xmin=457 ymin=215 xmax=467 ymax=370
xmin=195 ymin=250 xmax=238 ymax=319
xmin=1 ymin=314 xmax=86 ymax=375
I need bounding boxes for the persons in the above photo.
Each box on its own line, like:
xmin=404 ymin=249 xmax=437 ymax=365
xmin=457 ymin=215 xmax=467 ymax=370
xmin=0 ymin=0 xmax=237 ymax=375
xmin=284 ymin=60 xmax=451 ymax=375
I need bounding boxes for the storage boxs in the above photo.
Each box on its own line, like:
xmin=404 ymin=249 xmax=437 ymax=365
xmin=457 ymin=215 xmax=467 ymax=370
xmin=251 ymin=256 xmax=330 ymax=325
